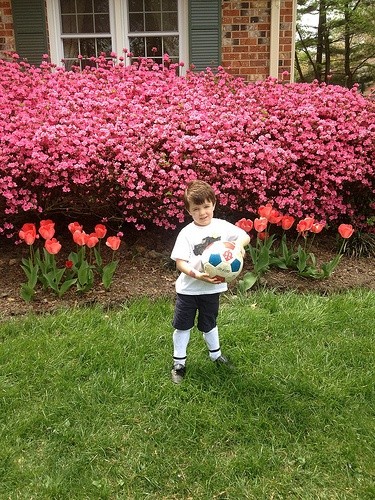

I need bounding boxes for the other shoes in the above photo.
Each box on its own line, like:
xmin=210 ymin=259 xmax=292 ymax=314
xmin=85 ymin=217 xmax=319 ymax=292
xmin=171 ymin=366 xmax=185 ymax=382
xmin=215 ymin=356 xmax=226 ymax=365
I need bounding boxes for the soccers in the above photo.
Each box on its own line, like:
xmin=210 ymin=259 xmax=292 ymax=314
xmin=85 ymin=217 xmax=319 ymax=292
xmin=200 ymin=238 xmax=243 ymax=281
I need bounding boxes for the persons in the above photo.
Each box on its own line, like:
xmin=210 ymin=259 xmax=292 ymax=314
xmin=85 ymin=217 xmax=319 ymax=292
xmin=171 ymin=180 xmax=250 ymax=384
